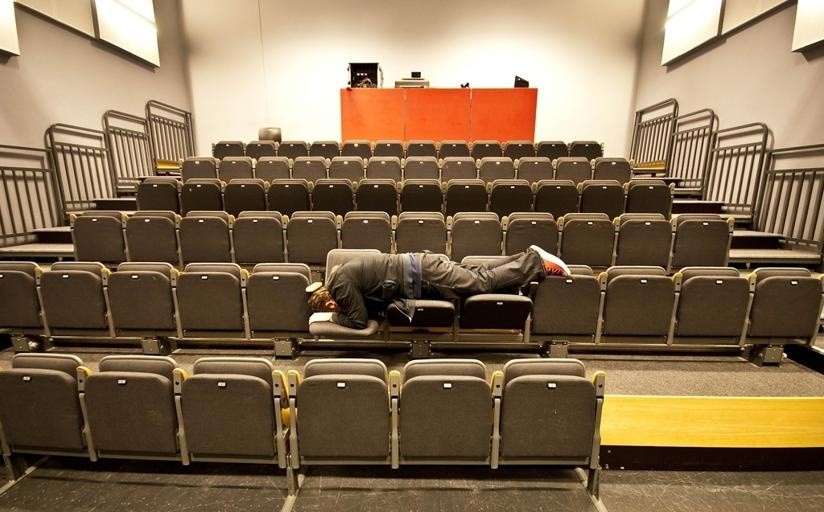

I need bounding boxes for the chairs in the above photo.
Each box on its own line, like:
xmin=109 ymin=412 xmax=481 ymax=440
xmin=258 ymin=126 xmax=282 ymax=145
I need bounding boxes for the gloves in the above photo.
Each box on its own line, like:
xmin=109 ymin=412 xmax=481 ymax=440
xmin=308 ymin=311 xmax=336 ymax=325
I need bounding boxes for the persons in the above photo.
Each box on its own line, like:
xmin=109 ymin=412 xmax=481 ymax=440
xmin=307 ymin=243 xmax=573 ymax=329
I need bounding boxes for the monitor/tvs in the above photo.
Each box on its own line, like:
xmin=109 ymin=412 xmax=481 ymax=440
xmin=515 ymin=76 xmax=529 ymax=88
xmin=394 ymin=81 xmax=429 ymax=88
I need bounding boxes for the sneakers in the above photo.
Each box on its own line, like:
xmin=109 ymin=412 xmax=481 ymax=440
xmin=530 ymin=245 xmax=571 ymax=276
